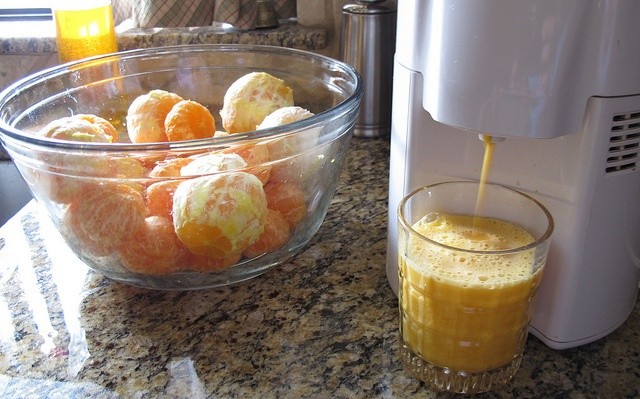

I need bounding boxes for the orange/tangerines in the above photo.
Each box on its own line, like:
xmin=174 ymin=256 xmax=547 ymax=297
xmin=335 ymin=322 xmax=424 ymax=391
xmin=31 ymin=72 xmax=322 ymax=275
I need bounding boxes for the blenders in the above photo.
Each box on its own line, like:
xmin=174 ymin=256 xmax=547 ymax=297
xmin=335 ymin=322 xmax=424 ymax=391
xmin=386 ymin=1 xmax=640 ymax=350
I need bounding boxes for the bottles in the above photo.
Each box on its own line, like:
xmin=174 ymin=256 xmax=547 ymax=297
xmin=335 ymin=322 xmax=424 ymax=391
xmin=339 ymin=0 xmax=397 ymax=138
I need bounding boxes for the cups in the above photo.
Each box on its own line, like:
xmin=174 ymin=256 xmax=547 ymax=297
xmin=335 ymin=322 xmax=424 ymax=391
xmin=397 ymin=180 xmax=555 ymax=394
xmin=51 ymin=0 xmax=118 ymax=65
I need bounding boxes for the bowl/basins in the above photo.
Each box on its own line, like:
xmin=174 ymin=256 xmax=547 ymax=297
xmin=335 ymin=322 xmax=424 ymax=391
xmin=0 ymin=44 xmax=363 ymax=290
